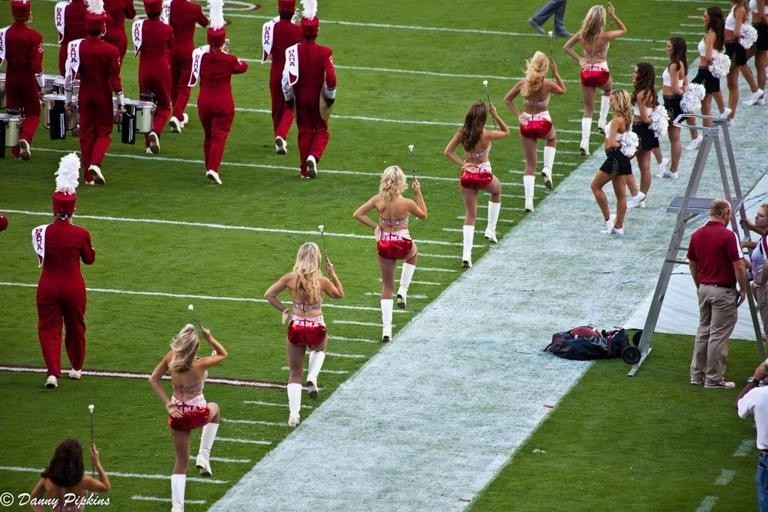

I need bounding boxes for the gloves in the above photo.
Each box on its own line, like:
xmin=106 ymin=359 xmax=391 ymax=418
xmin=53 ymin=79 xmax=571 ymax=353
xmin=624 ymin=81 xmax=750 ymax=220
xmin=329 ymin=98 xmax=335 ymax=107
xmin=287 ymin=99 xmax=293 ymax=108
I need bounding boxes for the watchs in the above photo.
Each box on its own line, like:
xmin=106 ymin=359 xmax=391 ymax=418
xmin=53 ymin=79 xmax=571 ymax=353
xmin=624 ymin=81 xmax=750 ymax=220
xmin=747 ymin=376 xmax=759 ymax=386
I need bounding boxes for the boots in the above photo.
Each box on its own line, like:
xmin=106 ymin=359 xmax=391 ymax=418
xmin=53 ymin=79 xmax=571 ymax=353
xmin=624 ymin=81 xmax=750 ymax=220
xmin=598 ymin=96 xmax=610 ymax=135
xmin=306 ymin=350 xmax=325 ymax=399
xmin=580 ymin=117 xmax=592 ymax=156
xmin=484 ymin=201 xmax=501 ymax=244
xmin=381 ymin=298 xmax=393 ymax=342
xmin=541 ymin=146 xmax=556 ymax=189
xmin=287 ymin=383 xmax=303 ymax=427
xmin=398 ymin=263 xmax=415 ymax=308
xmin=462 ymin=225 xmax=474 ymax=268
xmin=171 ymin=473 xmax=186 ymax=512
xmin=195 ymin=422 xmax=221 ymax=475
xmin=523 ymin=174 xmax=535 ymax=212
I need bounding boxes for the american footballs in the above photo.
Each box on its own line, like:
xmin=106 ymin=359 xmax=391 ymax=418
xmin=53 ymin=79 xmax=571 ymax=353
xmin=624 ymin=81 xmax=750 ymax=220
xmin=571 ymin=327 xmax=597 ymax=338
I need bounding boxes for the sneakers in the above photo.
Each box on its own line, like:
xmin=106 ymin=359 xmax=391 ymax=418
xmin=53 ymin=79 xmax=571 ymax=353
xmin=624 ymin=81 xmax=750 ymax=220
xmin=275 ymin=136 xmax=287 ymax=154
xmin=207 ymin=170 xmax=222 ymax=184
xmin=20 ymin=139 xmax=32 ymax=161
xmin=146 ymin=147 xmax=154 ymax=154
xmin=687 ymin=135 xmax=703 ymax=151
xmin=704 ymin=379 xmax=736 ymax=389
xmin=601 ymin=226 xmax=624 ymax=236
xmin=745 ymin=88 xmax=765 ymax=106
xmin=45 ymin=376 xmax=59 ymax=389
xmin=627 ymin=191 xmax=645 ymax=208
xmin=665 ymin=169 xmax=678 ymax=179
xmin=69 ymin=369 xmax=83 ymax=380
xmin=719 ymin=108 xmax=735 ymax=126
xmin=606 ymin=214 xmax=616 ymax=234
xmin=301 ymin=154 xmax=317 ymax=178
xmin=179 ymin=113 xmax=188 ymax=129
xmin=169 ymin=116 xmax=181 ymax=134
xmin=691 ymin=377 xmax=706 ymax=384
xmin=89 ymin=165 xmax=105 ymax=185
xmin=86 ymin=179 xmax=95 ymax=186
xmin=656 ymin=158 xmax=669 ymax=178
xmin=148 ymin=131 xmax=160 ymax=154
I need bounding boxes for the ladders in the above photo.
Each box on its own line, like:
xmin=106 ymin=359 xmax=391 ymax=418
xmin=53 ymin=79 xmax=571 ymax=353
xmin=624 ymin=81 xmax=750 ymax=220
xmin=622 ymin=111 xmax=764 ymax=377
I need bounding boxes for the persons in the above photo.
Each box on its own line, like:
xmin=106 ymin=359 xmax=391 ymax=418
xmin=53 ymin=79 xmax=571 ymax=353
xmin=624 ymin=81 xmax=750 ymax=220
xmin=739 ymin=218 xmax=766 ymax=249
xmin=54 ymin=0 xmax=89 ymax=79
xmin=686 ymin=197 xmax=746 ymax=389
xmin=133 ymin=0 xmax=175 ymax=155
xmin=663 ymin=37 xmax=704 ymax=180
xmin=717 ymin=0 xmax=764 ymax=128
xmin=443 ymin=100 xmax=510 ymax=269
xmin=563 ymin=2 xmax=627 ymax=156
xmin=31 ymin=192 xmax=97 ymax=389
xmin=28 ymin=439 xmax=111 ymax=512
xmin=0 ymin=0 xmax=44 ymax=161
xmin=148 ymin=324 xmax=228 ymax=512
xmin=263 ymin=0 xmax=302 ymax=156
xmin=285 ymin=16 xmax=337 ymax=179
xmin=164 ymin=0 xmax=211 ymax=134
xmin=192 ymin=27 xmax=249 ymax=186
xmin=748 ymin=0 xmax=768 ymax=107
xmin=732 ymin=357 xmax=768 ymax=512
xmin=742 ymin=204 xmax=768 ymax=337
xmin=503 ymin=51 xmax=567 ymax=214
xmin=263 ymin=242 xmax=344 ymax=428
xmin=528 ymin=0 xmax=574 ymax=37
xmin=689 ymin=6 xmax=732 ymax=136
xmin=591 ymin=89 xmax=633 ymax=235
xmin=352 ymin=165 xmax=428 ymax=342
xmin=64 ymin=11 xmax=127 ymax=186
xmin=97 ymin=0 xmax=137 ymax=91
xmin=627 ymin=62 xmax=669 ymax=208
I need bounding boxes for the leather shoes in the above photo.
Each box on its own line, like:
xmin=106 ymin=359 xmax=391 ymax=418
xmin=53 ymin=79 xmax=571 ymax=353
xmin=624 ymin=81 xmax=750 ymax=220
xmin=555 ymin=31 xmax=574 ymax=37
xmin=530 ymin=17 xmax=545 ymax=34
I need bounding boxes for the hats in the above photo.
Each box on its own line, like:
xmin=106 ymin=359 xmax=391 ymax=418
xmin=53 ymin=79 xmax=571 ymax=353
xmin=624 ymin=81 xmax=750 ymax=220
xmin=300 ymin=0 xmax=319 ymax=36
xmin=52 ymin=153 xmax=80 ymax=215
xmin=84 ymin=0 xmax=105 ymax=31
xmin=144 ymin=0 xmax=162 ymax=16
xmin=12 ymin=0 xmax=31 ymax=21
xmin=207 ymin=0 xmax=226 ymax=45
xmin=279 ymin=0 xmax=296 ymax=14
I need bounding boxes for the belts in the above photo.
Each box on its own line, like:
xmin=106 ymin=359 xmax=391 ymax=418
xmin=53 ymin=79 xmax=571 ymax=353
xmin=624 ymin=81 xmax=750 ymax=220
xmin=703 ymin=283 xmax=736 ymax=288
xmin=763 ymin=449 xmax=768 ymax=454
